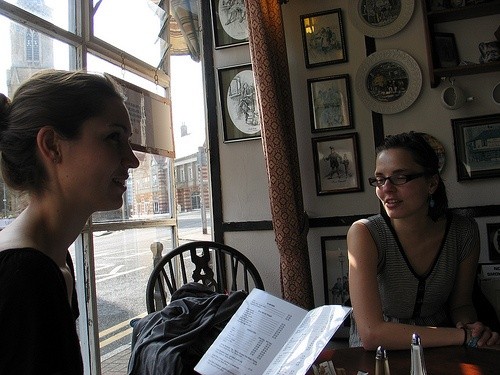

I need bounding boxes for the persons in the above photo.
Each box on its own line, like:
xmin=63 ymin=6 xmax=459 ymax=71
xmin=0 ymin=68 xmax=141 ymax=375
xmin=346 ymin=130 xmax=500 ymax=353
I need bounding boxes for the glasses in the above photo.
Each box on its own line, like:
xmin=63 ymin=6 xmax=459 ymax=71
xmin=368 ymin=172 xmax=432 ymax=186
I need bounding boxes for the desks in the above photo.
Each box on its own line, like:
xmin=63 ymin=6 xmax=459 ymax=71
xmin=303 ymin=332 xmax=500 ymax=374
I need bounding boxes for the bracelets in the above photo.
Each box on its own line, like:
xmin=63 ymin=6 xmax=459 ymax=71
xmin=460 ymin=328 xmax=468 ymax=346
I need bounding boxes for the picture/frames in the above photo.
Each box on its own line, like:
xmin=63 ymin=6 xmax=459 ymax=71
xmin=299 ymin=7 xmax=348 ymax=69
xmin=310 ymin=132 xmax=364 ymax=197
xmin=430 ymin=31 xmax=459 ymax=68
xmin=215 ymin=62 xmax=262 ymax=144
xmin=321 ymin=235 xmax=347 ymax=325
xmin=210 ymin=0 xmax=250 ymax=50
xmin=307 ymin=73 xmax=354 ymax=134
xmin=450 ymin=113 xmax=500 ymax=182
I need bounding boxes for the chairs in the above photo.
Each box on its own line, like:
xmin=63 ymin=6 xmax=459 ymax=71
xmin=147 ymin=240 xmax=265 ymax=313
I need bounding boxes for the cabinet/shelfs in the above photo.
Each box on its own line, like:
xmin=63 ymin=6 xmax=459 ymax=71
xmin=423 ymin=0 xmax=500 ymax=89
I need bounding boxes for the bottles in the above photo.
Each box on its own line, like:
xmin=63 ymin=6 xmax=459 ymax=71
xmin=411 ymin=332 xmax=426 ymax=375
xmin=374 ymin=344 xmax=390 ymax=375
